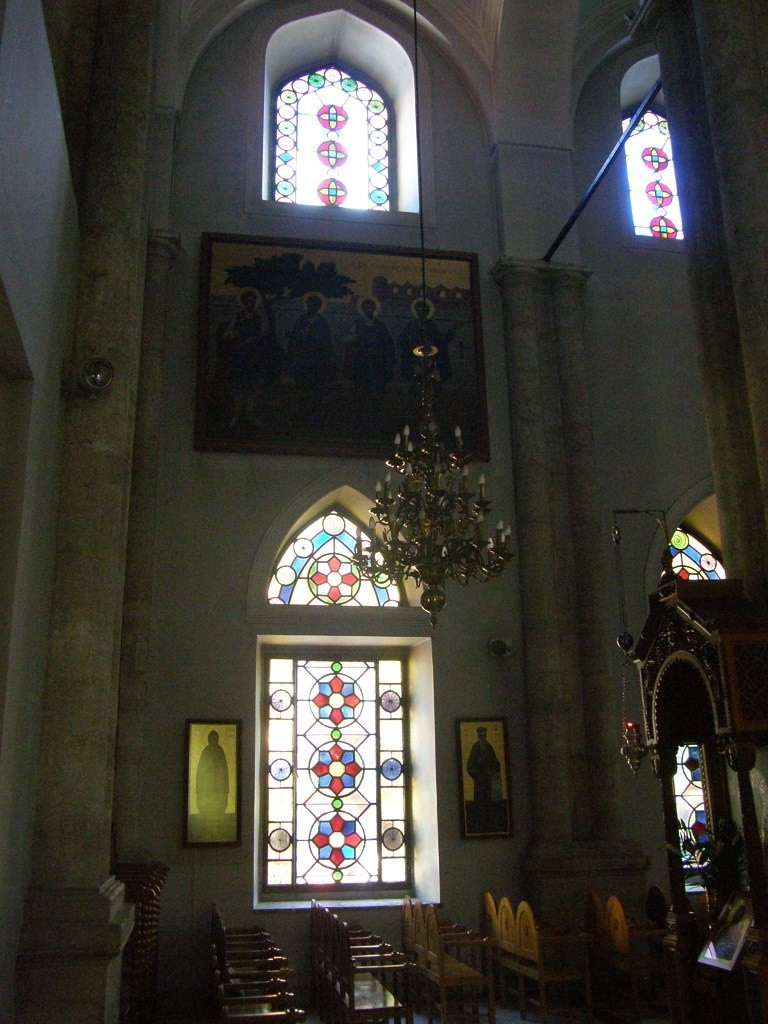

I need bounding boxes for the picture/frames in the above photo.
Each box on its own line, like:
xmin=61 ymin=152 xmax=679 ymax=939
xmin=193 ymin=232 xmax=491 ymax=463
xmin=697 ymin=894 xmax=754 ymax=971
xmin=454 ymin=715 xmax=517 ymax=841
xmin=183 ymin=717 xmax=243 ymax=849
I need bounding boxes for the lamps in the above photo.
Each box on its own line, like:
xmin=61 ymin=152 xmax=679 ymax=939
xmin=352 ymin=0 xmax=516 ymax=631
xmin=620 ymin=651 xmax=648 ymax=782
xmin=60 ymin=356 xmax=115 ymax=401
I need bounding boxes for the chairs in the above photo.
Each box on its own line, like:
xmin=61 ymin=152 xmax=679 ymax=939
xmin=205 ymin=902 xmax=307 ymax=1024
xmin=479 ymin=891 xmax=498 ymax=1006
xmin=581 ymin=890 xmax=651 ymax=1012
xmin=516 ymin=901 xmax=601 ymax=1024
xmin=604 ymin=895 xmax=675 ymax=1024
xmin=497 ymin=897 xmax=563 ymax=1009
xmin=423 ymin=903 xmax=495 ymax=1024
xmin=400 ymin=894 xmax=453 ymax=1002
xmin=411 ymin=903 xmax=469 ymax=1014
xmin=310 ymin=906 xmax=418 ymax=1024
